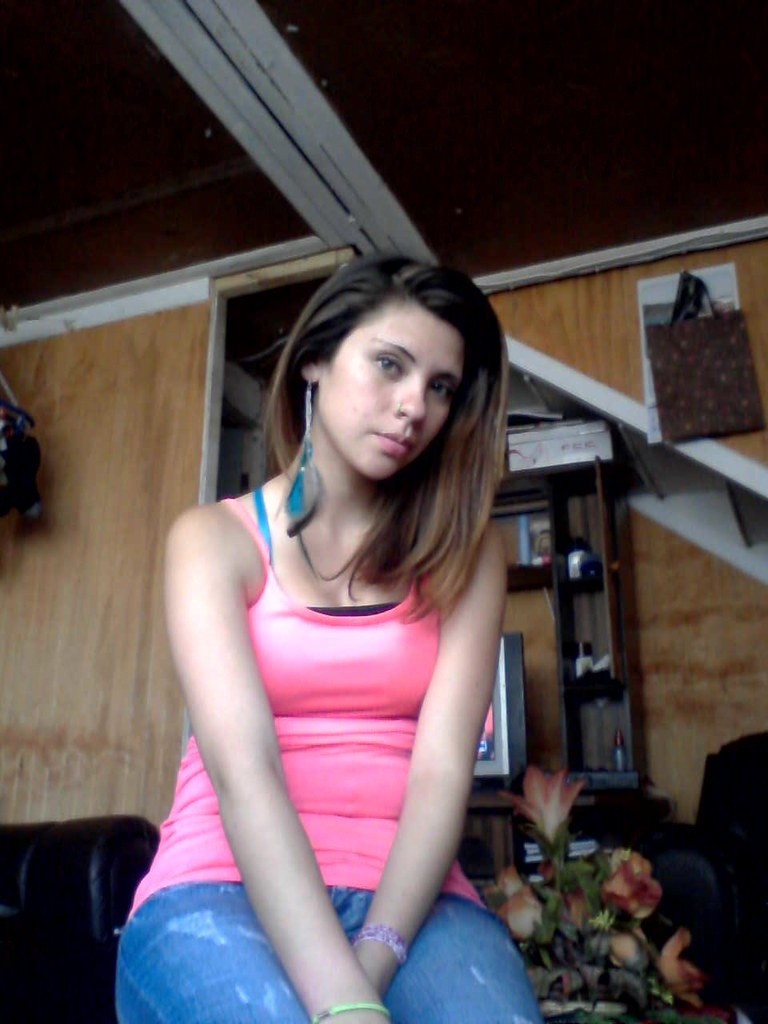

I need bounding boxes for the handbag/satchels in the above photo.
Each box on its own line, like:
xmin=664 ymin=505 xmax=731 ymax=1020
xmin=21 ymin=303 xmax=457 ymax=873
xmin=646 ymin=272 xmax=764 ymax=443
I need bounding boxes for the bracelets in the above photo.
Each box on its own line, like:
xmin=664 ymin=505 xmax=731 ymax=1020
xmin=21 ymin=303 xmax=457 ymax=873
xmin=352 ymin=924 xmax=407 ymax=964
xmin=311 ymin=1001 xmax=390 ymax=1024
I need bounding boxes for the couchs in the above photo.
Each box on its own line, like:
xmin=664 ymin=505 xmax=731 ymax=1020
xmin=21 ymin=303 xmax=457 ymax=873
xmin=1 ymin=813 xmax=162 ymax=1023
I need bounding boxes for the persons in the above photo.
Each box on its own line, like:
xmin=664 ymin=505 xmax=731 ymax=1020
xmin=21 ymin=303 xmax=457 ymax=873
xmin=114 ymin=254 xmax=548 ymax=1024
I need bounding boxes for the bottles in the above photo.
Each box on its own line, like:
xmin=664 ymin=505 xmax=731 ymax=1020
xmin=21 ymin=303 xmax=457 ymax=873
xmin=611 ymin=729 xmax=626 ymax=771
xmin=568 ymin=538 xmax=591 ymax=579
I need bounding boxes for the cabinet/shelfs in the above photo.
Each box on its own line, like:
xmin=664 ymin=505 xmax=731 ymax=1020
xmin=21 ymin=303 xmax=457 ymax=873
xmin=470 ymin=454 xmax=646 ymax=809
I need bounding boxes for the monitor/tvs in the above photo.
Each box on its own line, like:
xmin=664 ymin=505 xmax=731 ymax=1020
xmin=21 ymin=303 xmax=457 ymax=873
xmin=470 ymin=632 xmax=528 ymax=795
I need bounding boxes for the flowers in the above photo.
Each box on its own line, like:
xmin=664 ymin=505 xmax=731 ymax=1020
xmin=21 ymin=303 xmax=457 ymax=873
xmin=477 ymin=763 xmax=712 ymax=1024
